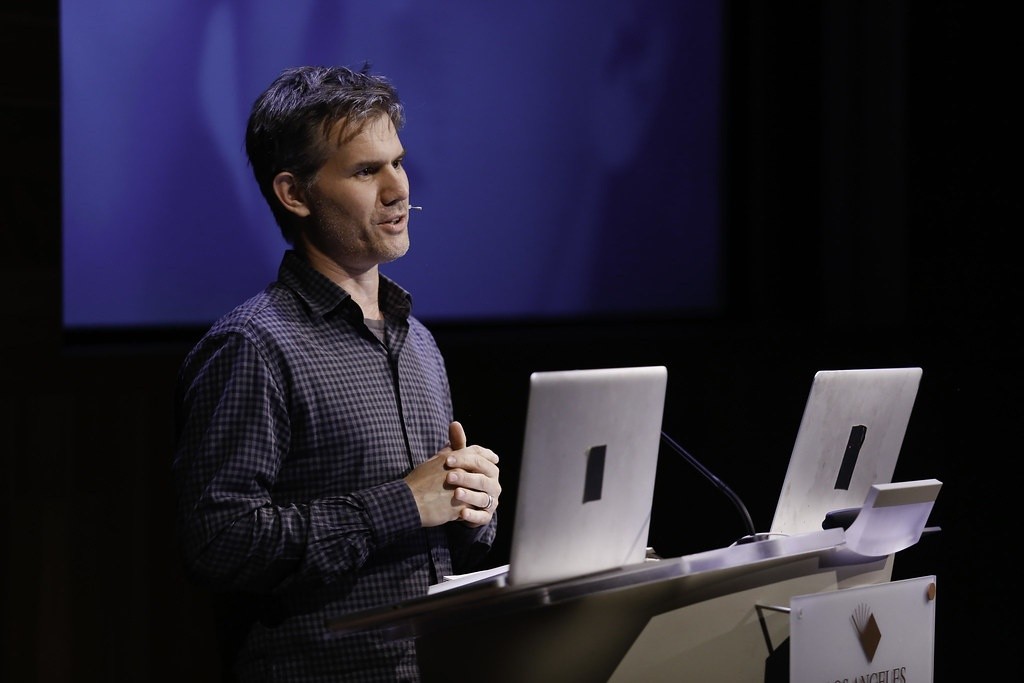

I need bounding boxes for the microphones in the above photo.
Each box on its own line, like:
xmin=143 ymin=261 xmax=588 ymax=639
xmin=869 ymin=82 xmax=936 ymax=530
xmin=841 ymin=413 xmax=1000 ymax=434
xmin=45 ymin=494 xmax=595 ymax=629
xmin=409 ymin=205 xmax=422 ymax=211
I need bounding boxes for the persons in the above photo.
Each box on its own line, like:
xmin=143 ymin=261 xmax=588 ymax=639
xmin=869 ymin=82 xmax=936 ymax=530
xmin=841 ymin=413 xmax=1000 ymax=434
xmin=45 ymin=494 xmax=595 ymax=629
xmin=173 ymin=65 xmax=503 ymax=683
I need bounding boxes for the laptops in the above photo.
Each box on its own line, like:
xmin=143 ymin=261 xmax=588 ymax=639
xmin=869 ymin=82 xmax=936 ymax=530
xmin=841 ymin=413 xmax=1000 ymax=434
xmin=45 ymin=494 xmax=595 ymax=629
xmin=325 ymin=366 xmax=668 ymax=628
xmin=768 ymin=368 xmax=923 ymax=541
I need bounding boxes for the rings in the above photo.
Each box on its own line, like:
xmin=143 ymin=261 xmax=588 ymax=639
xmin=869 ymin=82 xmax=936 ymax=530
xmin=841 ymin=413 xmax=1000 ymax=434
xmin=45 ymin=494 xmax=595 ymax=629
xmin=485 ymin=495 xmax=493 ymax=509
